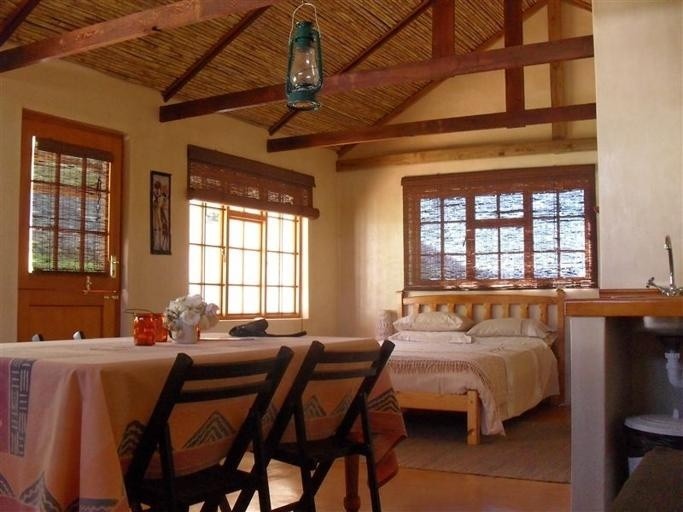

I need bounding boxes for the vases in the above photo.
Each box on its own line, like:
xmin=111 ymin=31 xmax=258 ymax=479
xmin=169 ymin=325 xmax=200 ymax=343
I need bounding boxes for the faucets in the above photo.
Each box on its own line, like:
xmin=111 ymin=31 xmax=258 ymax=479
xmin=664 ymin=235 xmax=675 ymax=287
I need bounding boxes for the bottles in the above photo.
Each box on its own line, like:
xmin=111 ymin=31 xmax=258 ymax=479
xmin=132 ymin=312 xmax=155 ymax=346
xmin=152 ymin=312 xmax=166 ymax=342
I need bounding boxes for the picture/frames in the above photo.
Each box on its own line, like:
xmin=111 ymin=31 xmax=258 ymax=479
xmin=146 ymin=168 xmax=172 ymax=257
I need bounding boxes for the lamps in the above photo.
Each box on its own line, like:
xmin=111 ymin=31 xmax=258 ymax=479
xmin=373 ymin=309 xmax=396 ymax=344
xmin=281 ymin=0 xmax=327 ymax=112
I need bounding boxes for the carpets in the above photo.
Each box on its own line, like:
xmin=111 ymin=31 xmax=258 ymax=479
xmin=393 ymin=414 xmax=574 ymax=485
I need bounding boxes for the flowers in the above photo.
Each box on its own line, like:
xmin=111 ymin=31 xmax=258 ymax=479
xmin=160 ymin=294 xmax=221 ymax=333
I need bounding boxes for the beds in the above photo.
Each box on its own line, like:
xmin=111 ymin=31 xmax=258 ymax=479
xmin=371 ymin=287 xmax=566 ymax=447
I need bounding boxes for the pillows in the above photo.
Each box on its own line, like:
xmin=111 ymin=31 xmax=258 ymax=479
xmin=389 ymin=331 xmax=472 ymax=345
xmin=466 ymin=316 xmax=557 ymax=342
xmin=392 ymin=313 xmax=477 ymax=333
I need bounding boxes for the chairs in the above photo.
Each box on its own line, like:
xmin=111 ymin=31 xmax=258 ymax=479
xmin=121 ymin=343 xmax=295 ymax=512
xmin=217 ymin=333 xmax=396 ymax=512
xmin=32 ymin=331 xmax=84 ymax=341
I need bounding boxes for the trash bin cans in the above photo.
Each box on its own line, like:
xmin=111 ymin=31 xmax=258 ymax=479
xmin=623 ymin=414 xmax=683 ymax=479
